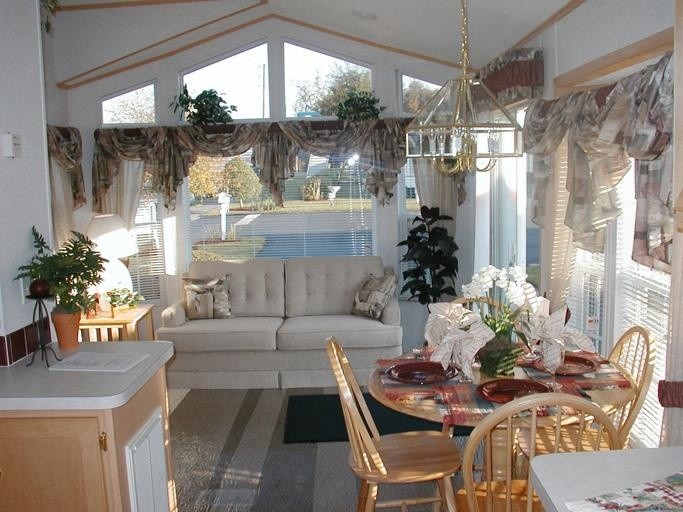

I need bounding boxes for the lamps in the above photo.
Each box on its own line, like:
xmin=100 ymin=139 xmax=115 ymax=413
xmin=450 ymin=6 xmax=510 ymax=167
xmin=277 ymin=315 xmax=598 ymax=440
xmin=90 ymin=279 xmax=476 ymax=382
xmin=85 ymin=216 xmax=138 ymax=313
xmin=400 ymin=1 xmax=526 ymax=175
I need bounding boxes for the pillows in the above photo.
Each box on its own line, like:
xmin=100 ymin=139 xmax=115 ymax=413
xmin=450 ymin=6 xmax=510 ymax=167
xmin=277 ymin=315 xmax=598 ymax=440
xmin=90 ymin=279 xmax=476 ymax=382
xmin=349 ymin=270 xmax=399 ymax=322
xmin=182 ymin=274 xmax=236 ymax=322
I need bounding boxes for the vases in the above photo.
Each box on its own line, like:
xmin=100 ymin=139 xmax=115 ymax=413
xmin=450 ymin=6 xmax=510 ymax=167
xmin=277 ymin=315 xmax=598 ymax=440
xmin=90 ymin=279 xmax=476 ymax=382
xmin=479 ymin=344 xmax=519 ymax=377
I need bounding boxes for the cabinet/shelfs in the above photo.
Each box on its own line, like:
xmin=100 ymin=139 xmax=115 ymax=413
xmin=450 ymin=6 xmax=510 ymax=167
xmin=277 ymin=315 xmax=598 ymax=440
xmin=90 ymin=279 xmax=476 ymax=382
xmin=0 ymin=337 xmax=183 ymax=512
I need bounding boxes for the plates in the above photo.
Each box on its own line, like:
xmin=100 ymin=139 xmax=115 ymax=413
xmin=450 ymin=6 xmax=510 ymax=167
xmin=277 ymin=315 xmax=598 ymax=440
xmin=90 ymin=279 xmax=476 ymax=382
xmin=386 ymin=361 xmax=460 ymax=384
xmin=474 ymin=377 xmax=552 ymax=403
xmin=534 ymin=354 xmax=597 ymax=377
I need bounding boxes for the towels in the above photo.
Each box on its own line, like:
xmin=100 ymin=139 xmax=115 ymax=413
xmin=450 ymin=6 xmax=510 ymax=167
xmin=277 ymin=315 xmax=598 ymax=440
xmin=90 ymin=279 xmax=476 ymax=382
xmin=421 ymin=301 xmax=496 ymax=384
xmin=527 ymin=295 xmax=596 ymax=374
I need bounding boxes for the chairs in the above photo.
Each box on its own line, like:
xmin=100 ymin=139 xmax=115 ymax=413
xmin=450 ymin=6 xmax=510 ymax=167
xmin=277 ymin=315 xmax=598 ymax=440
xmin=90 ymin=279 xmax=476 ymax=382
xmin=449 ymin=297 xmax=523 ymax=344
xmin=458 ymin=393 xmax=624 ymax=511
xmin=320 ymin=334 xmax=465 ymax=511
xmin=509 ymin=324 xmax=657 ymax=477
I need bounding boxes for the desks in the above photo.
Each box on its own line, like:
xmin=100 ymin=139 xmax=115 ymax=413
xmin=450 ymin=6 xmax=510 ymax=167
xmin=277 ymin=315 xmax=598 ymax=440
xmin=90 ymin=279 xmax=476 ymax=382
xmin=76 ymin=301 xmax=153 ymax=344
xmin=525 ymin=444 xmax=682 ymax=511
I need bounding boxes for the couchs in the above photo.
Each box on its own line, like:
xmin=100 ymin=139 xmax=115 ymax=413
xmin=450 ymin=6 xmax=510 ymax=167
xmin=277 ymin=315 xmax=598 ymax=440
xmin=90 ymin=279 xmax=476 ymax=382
xmin=154 ymin=252 xmax=405 ymax=355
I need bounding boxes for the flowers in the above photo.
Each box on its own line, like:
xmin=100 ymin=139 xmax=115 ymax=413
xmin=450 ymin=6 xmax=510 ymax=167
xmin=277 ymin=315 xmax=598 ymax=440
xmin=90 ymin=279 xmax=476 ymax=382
xmin=461 ymin=261 xmax=528 ymax=338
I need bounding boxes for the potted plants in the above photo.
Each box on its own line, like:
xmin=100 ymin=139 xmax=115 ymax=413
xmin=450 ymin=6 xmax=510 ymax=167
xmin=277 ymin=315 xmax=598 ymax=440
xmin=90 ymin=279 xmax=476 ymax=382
xmin=14 ymin=222 xmax=112 ymax=352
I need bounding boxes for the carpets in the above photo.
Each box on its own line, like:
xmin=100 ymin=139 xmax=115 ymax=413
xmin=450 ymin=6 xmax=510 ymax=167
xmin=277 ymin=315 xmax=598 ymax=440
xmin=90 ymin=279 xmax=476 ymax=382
xmin=280 ymin=391 xmax=478 ymax=448
